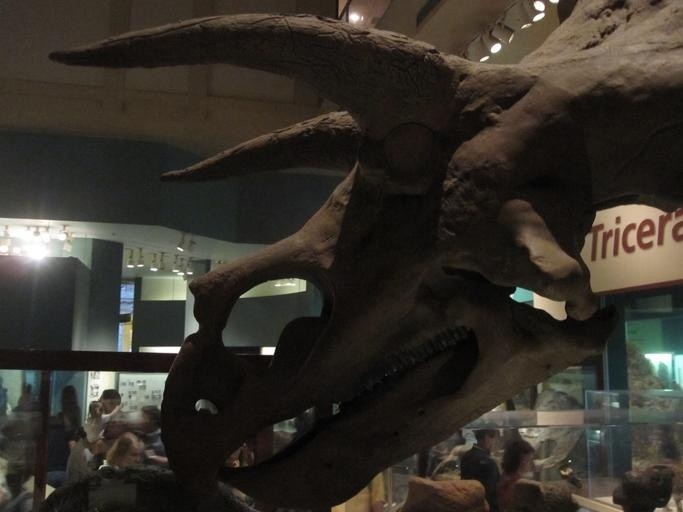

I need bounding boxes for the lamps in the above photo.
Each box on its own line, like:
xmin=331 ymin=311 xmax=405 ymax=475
xmin=125 ymin=232 xmax=196 ymax=276
xmin=467 ymin=0 xmax=547 ymax=62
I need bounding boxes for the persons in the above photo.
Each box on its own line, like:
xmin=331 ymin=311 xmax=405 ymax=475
xmin=498 ymin=439 xmax=535 ymax=511
xmin=1 ymin=383 xmax=168 ymax=511
xmin=460 ymin=428 xmax=500 ymax=511
xmin=331 ymin=471 xmax=386 ymax=512
